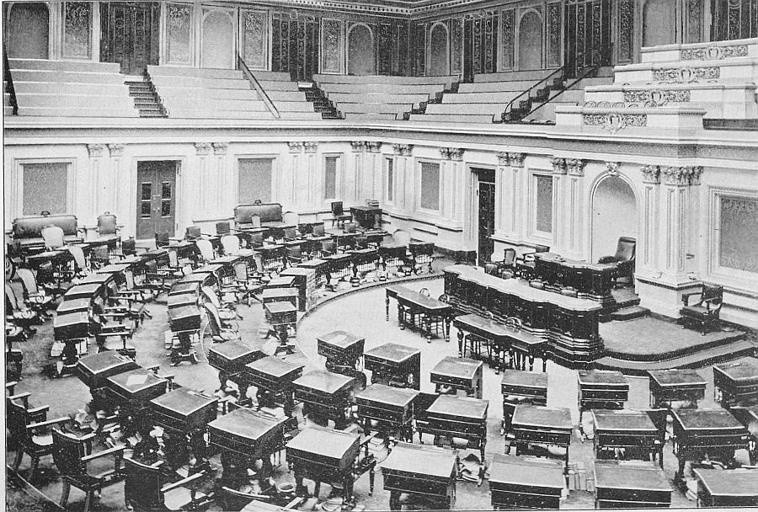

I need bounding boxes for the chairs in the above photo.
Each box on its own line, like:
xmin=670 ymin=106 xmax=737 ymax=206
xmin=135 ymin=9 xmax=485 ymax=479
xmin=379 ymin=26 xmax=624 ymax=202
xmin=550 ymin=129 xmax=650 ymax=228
xmin=493 ymin=247 xmax=516 ymax=276
xmin=680 ymin=283 xmax=724 ymax=335
xmin=598 ymin=237 xmax=636 ymax=288
xmin=5 ymin=202 xmax=493 ymax=378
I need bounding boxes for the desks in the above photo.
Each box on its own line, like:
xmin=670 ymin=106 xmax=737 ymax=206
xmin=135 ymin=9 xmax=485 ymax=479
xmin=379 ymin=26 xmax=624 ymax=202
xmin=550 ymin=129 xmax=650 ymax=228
xmin=533 ymin=252 xmax=616 ymax=303
xmin=6 ymin=327 xmax=758 ymax=509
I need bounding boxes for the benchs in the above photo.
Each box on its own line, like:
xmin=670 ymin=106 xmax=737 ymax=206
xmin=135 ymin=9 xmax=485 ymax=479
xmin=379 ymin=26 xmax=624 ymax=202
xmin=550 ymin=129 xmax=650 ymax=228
xmin=6 ymin=38 xmax=622 ymax=126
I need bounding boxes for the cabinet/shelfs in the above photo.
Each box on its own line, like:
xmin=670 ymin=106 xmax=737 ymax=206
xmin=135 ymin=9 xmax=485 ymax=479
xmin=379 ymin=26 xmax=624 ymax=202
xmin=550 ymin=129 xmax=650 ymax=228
xmin=440 ymin=263 xmax=604 ymax=366
